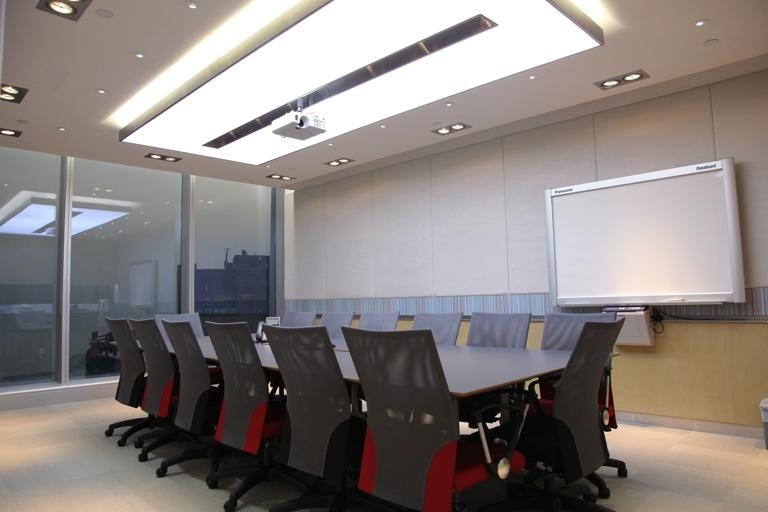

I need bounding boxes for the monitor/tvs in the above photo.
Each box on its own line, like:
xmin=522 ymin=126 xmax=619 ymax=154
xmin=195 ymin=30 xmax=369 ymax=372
xmin=272 ymin=111 xmax=326 ymax=140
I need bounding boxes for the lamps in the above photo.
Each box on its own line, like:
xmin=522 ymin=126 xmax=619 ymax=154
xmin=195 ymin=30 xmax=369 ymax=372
xmin=0 ymin=197 xmax=133 ymax=239
xmin=120 ymin=0 xmax=604 ymax=165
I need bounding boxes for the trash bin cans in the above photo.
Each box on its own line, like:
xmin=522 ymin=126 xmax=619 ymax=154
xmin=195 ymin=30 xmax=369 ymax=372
xmin=758 ymin=397 xmax=768 ymax=450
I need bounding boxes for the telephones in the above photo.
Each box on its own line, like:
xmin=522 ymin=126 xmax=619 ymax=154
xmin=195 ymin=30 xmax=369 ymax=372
xmin=256 ymin=316 xmax=281 ymax=343
